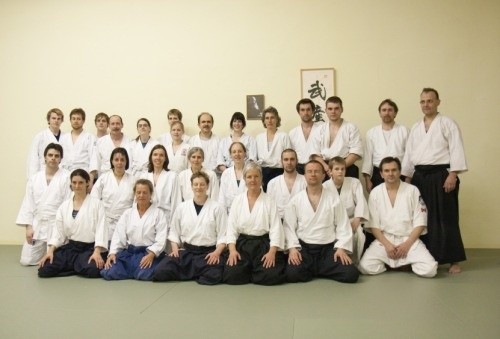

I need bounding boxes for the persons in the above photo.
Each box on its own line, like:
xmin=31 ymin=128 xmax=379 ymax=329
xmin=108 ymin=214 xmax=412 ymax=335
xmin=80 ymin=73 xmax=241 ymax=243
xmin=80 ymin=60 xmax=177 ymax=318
xmin=16 ymin=87 xmax=468 ymax=286
